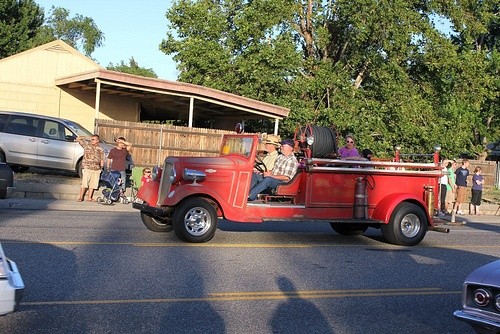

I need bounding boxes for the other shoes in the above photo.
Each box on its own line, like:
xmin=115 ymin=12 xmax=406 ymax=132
xmin=77 ymin=198 xmax=84 ymax=202
xmin=457 ymin=211 xmax=465 ymax=215
xmin=86 ymin=198 xmax=96 ymax=202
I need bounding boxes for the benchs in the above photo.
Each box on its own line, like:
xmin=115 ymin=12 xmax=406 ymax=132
xmin=271 ymin=148 xmax=311 ymax=196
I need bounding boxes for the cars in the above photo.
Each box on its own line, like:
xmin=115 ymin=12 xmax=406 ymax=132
xmin=452 ymin=259 xmax=500 ymax=334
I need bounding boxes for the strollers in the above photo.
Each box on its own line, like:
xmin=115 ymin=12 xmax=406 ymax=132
xmin=97 ymin=169 xmax=129 ymax=205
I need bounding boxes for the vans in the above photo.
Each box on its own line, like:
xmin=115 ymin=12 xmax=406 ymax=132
xmin=0 ymin=111 xmax=134 ymax=179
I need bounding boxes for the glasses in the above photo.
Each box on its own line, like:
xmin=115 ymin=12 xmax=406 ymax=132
xmin=346 ymin=141 xmax=353 ymax=144
xmin=144 ymin=171 xmax=151 ymax=173
xmin=91 ymin=139 xmax=96 ymax=140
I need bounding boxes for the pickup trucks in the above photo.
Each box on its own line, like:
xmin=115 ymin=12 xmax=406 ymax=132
xmin=132 ymin=122 xmax=450 ymax=246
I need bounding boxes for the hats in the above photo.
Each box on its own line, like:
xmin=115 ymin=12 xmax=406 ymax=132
xmin=261 ymin=134 xmax=280 ymax=148
xmin=279 ymin=137 xmax=294 ymax=148
xmin=362 ymin=148 xmax=371 ymax=158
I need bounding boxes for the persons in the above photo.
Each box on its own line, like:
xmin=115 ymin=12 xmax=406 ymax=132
xmin=253 ymin=134 xmax=281 ymax=174
xmin=248 ymin=139 xmax=299 ymax=201
xmin=76 ymin=135 xmax=104 ymax=202
xmin=106 ymin=137 xmax=132 ymax=194
xmin=469 ymin=167 xmax=485 ymax=215
xmin=438 ymin=159 xmax=470 ymax=215
xmin=141 ymin=168 xmax=153 ymax=186
xmin=332 ymin=135 xmax=374 ymax=169
